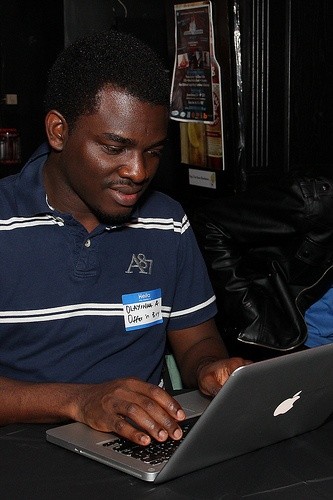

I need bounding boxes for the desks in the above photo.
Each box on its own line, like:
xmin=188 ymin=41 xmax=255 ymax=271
xmin=0 ymin=388 xmax=333 ymax=500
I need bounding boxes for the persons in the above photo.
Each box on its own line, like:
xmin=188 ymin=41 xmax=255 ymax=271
xmin=0 ymin=28 xmax=257 ymax=446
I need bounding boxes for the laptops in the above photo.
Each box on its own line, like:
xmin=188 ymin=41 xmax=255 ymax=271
xmin=47 ymin=343 xmax=332 ymax=481
